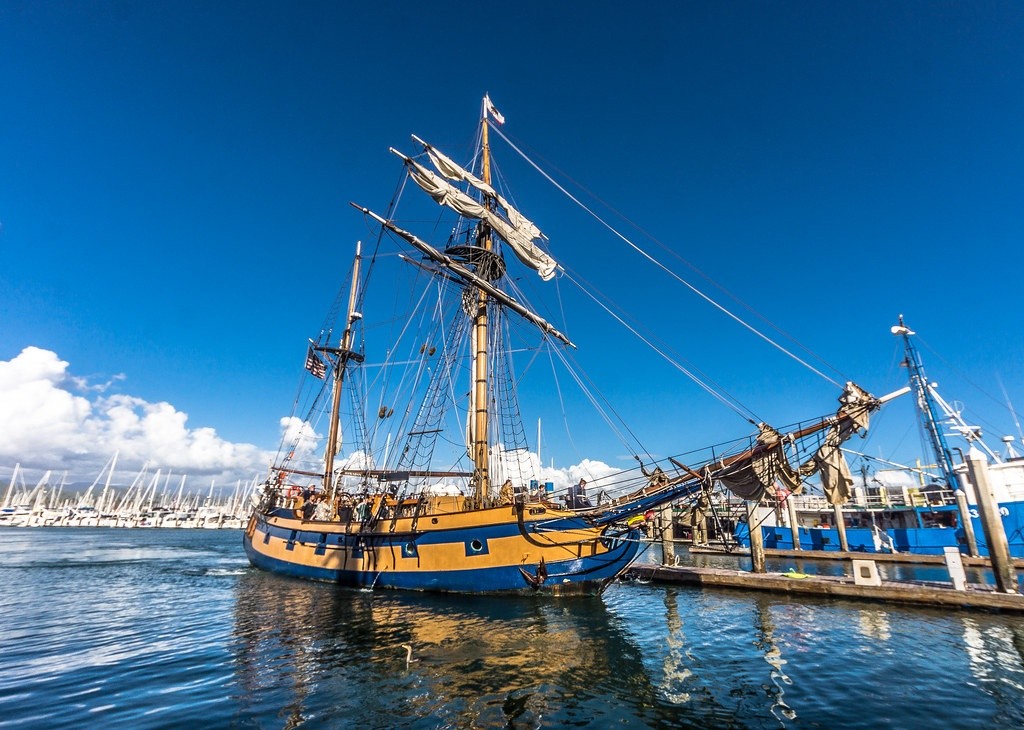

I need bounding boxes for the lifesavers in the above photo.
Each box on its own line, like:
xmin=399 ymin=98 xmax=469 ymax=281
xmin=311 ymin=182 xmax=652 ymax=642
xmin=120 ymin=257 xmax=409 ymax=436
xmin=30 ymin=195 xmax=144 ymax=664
xmin=288 ymin=487 xmax=301 ymax=497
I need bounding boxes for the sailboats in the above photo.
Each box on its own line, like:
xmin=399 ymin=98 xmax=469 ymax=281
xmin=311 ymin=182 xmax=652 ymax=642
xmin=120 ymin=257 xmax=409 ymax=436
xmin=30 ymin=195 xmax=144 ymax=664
xmin=244 ymin=90 xmax=908 ymax=600
xmin=0 ymin=457 xmax=261 ymax=529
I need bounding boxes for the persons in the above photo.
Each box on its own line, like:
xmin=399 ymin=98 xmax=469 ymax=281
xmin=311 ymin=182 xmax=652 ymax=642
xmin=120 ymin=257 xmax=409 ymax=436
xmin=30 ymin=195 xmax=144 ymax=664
xmin=919 ymin=478 xmax=946 ymax=505
xmin=499 ymin=479 xmax=586 ymax=505
xmin=303 ymin=495 xmax=331 ymax=521
